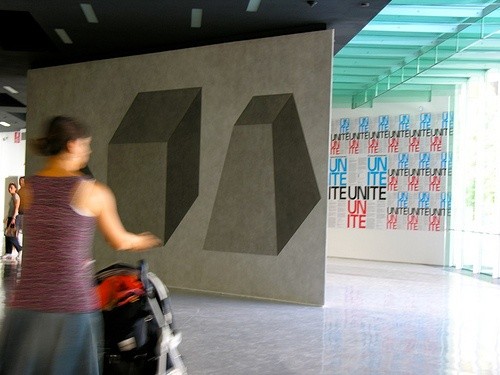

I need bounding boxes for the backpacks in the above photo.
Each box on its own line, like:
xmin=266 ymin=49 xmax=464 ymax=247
xmin=4 ymin=223 xmax=18 ymax=238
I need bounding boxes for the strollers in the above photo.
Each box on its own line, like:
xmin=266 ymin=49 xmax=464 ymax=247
xmin=94 ymin=259 xmax=188 ymax=375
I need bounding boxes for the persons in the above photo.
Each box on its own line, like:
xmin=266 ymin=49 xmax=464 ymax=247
xmin=12 ymin=176 xmax=25 ymax=251
xmin=0 ymin=114 xmax=164 ymax=375
xmin=0 ymin=182 xmax=23 ymax=261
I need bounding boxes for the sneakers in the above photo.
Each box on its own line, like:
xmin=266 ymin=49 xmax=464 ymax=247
xmin=16 ymin=250 xmax=23 ymax=261
xmin=1 ymin=251 xmax=12 ymax=260
